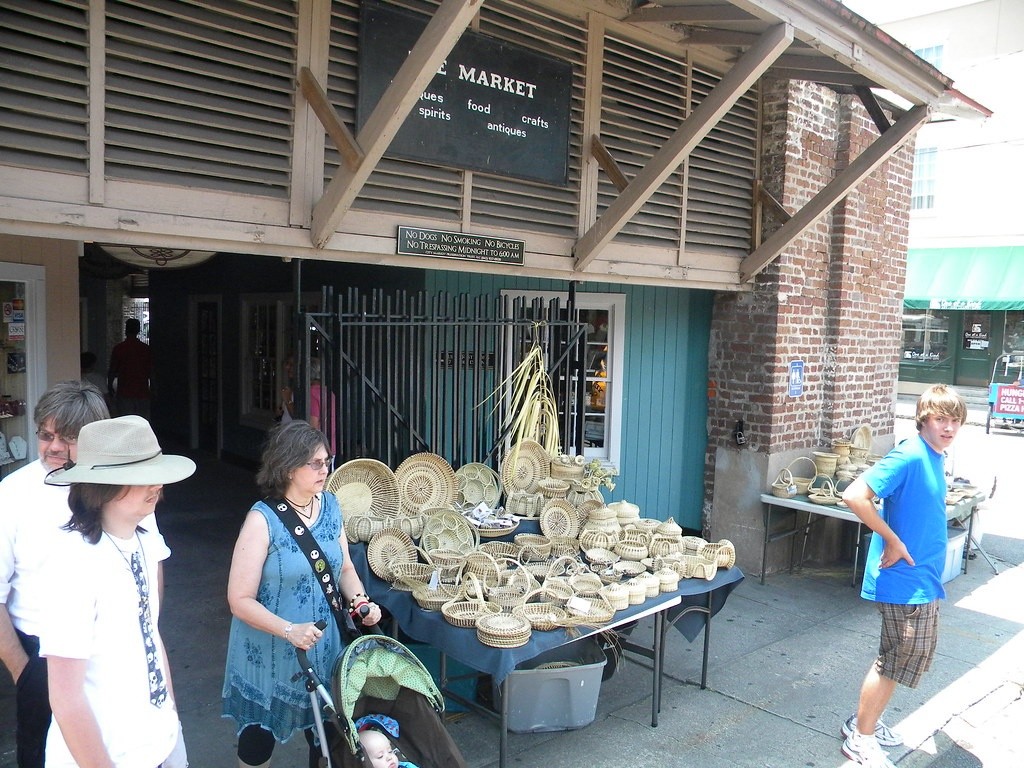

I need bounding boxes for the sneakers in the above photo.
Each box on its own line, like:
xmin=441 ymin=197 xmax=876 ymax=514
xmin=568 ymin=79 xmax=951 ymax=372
xmin=841 ymin=731 xmax=897 ymax=768
xmin=840 ymin=713 xmax=903 ymax=746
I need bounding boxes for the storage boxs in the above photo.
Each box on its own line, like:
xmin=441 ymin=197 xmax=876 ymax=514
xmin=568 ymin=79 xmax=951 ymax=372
xmin=863 ymin=508 xmax=985 ymax=585
xmin=404 ymin=644 xmax=476 ymax=713
xmin=491 ymin=639 xmax=607 ymax=733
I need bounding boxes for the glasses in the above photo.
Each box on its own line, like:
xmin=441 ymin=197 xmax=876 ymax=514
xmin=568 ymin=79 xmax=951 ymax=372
xmin=304 ymin=455 xmax=333 ymax=471
xmin=36 ymin=428 xmax=80 ymax=444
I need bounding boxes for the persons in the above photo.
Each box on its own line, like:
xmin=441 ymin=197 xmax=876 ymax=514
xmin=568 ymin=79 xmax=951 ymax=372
xmin=82 ymin=352 xmax=109 ymax=396
xmin=275 ymin=355 xmax=335 ymax=471
xmin=841 ymin=385 xmax=968 ymax=768
xmin=222 ymin=420 xmax=382 ymax=768
xmin=36 ymin=416 xmax=189 ymax=768
xmin=107 ymin=319 xmax=154 ymax=421
xmin=359 ymin=730 xmax=417 ymax=768
xmin=0 ymin=380 xmax=108 ymax=768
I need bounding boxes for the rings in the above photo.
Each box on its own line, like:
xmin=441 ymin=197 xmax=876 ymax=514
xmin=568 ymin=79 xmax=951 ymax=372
xmin=309 ymin=642 xmax=314 ymax=647
xmin=311 ymin=636 xmax=317 ymax=643
xmin=371 ymin=604 xmax=376 ymax=610
xmin=281 ymin=388 xmax=285 ymax=392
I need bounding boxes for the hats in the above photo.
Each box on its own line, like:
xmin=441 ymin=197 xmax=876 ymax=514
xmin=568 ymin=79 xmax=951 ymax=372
xmin=50 ymin=415 xmax=197 ymax=486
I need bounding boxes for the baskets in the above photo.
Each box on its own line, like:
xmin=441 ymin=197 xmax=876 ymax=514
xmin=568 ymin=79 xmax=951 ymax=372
xmin=326 ymin=437 xmax=736 ymax=648
xmin=772 ymin=425 xmax=978 ymax=510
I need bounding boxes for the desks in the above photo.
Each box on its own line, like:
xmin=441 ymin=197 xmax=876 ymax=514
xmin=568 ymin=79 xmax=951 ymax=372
xmin=344 ymin=517 xmax=748 ymax=768
xmin=760 ymin=493 xmax=986 ymax=588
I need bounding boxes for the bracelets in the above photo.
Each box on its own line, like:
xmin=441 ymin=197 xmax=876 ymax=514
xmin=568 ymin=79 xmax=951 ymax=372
xmin=348 ymin=593 xmax=370 ymax=618
xmin=286 ymin=400 xmax=293 ymax=405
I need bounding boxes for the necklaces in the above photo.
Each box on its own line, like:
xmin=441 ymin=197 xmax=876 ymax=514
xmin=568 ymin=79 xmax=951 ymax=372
xmin=103 ymin=530 xmax=151 ymax=610
xmin=286 ymin=497 xmax=313 ymax=523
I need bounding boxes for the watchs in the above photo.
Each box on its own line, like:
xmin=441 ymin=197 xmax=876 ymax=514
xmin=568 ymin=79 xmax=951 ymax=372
xmin=285 ymin=622 xmax=293 ymax=639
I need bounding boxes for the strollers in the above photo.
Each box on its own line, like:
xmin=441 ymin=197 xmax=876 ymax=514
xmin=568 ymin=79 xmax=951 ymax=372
xmin=290 ymin=606 xmax=469 ymax=768
